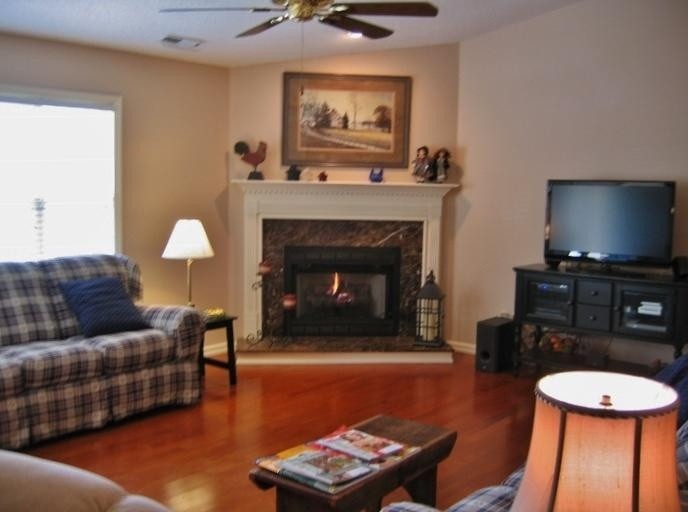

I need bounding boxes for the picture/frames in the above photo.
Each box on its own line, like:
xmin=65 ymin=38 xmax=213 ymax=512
xmin=278 ymin=69 xmax=413 ymax=168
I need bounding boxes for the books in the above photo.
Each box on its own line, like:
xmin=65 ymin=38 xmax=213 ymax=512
xmin=278 ymin=449 xmax=372 ymax=485
xmin=323 ymin=429 xmax=404 ymax=462
xmin=254 ymin=444 xmax=381 ymax=498
xmin=309 ymin=424 xmax=422 ymax=472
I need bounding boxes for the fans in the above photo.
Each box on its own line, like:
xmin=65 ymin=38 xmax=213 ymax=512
xmin=156 ymin=0 xmax=439 ymax=48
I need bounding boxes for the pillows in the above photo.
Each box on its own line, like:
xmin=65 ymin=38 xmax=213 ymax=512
xmin=59 ymin=277 xmax=150 ymax=338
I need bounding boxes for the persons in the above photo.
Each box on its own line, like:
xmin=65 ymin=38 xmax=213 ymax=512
xmin=427 ymin=147 xmax=453 ymax=183
xmin=410 ymin=145 xmax=430 ymax=184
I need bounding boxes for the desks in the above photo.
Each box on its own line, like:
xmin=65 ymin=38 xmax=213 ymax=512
xmin=510 ymin=262 xmax=687 ymax=378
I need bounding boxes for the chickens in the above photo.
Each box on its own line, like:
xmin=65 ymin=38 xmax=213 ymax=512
xmin=234 ymin=140 xmax=268 ymax=171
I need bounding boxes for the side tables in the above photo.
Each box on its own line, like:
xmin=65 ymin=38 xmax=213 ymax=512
xmin=202 ymin=315 xmax=239 ymax=385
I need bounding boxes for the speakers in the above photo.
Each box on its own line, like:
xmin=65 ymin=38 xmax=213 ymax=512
xmin=475 ymin=317 xmax=510 ymax=373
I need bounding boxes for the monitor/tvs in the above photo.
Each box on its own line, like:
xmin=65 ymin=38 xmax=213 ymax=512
xmin=545 ymin=179 xmax=675 ymax=267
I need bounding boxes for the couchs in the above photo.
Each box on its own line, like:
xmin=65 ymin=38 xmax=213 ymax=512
xmin=1 ymin=254 xmax=205 ymax=449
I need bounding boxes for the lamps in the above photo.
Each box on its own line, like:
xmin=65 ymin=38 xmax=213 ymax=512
xmin=507 ymin=364 xmax=683 ymax=512
xmin=159 ymin=217 xmax=215 ymax=311
xmin=412 ymin=271 xmax=447 ymax=348
